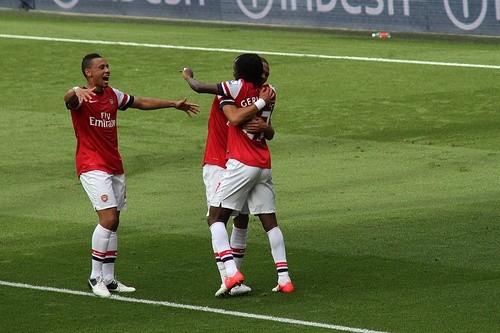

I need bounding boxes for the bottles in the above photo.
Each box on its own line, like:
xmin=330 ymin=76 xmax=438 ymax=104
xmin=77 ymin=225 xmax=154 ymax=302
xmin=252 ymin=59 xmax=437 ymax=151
xmin=372 ymin=32 xmax=391 ymax=39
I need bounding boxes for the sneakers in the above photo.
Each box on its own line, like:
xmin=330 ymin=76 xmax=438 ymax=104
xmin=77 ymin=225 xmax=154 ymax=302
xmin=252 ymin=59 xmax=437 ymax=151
xmin=87 ymin=275 xmax=137 ymax=298
xmin=272 ymin=277 xmax=296 ymax=293
xmin=215 ymin=273 xmax=244 ymax=297
xmin=230 ymin=285 xmax=252 ymax=295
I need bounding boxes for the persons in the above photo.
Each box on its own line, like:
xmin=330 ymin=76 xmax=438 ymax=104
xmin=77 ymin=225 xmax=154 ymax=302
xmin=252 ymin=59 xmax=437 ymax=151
xmin=64 ymin=53 xmax=201 ymax=297
xmin=183 ymin=53 xmax=294 ymax=298
